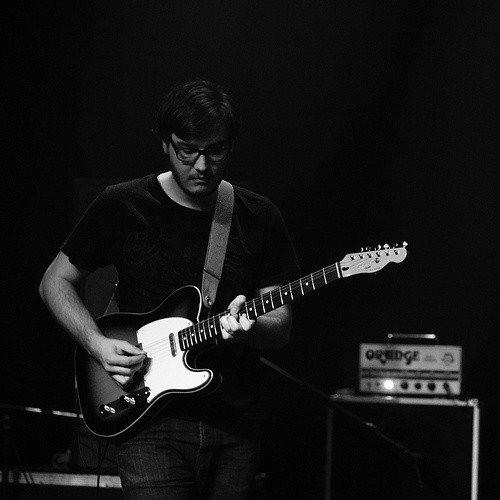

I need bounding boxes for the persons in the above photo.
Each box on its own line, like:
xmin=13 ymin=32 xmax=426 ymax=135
xmin=36 ymin=80 xmax=286 ymax=499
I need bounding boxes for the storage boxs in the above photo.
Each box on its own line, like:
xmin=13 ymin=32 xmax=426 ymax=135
xmin=326 ymin=387 xmax=482 ymax=500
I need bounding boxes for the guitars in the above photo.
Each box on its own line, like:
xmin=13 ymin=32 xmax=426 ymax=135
xmin=74 ymin=238 xmax=407 ymax=440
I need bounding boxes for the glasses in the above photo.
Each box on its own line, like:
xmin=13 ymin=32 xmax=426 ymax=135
xmin=168 ymin=137 xmax=234 ymax=164
